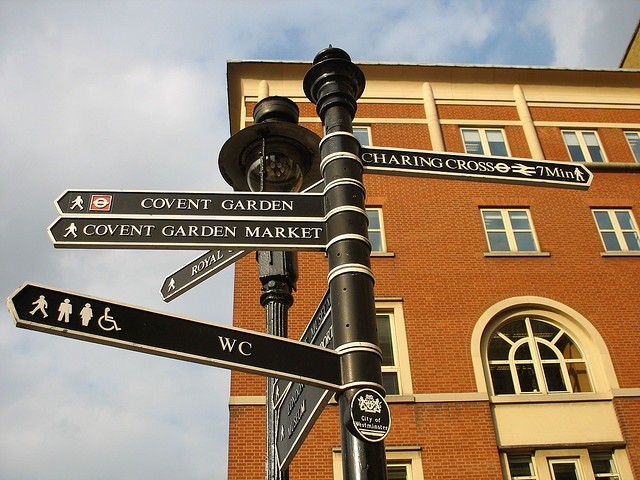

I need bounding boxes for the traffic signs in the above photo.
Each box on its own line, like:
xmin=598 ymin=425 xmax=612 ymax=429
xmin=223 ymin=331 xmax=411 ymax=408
xmin=271 ymin=289 xmax=332 ymax=410
xmin=215 ymin=310 xmax=336 ymax=475
xmin=159 ymin=178 xmax=326 ymax=303
xmin=48 ymin=216 xmax=326 ymax=250
xmin=362 ymin=146 xmax=595 ymax=190
xmin=9 ymin=281 xmax=339 ymax=397
xmin=53 ymin=189 xmax=327 ymax=218
